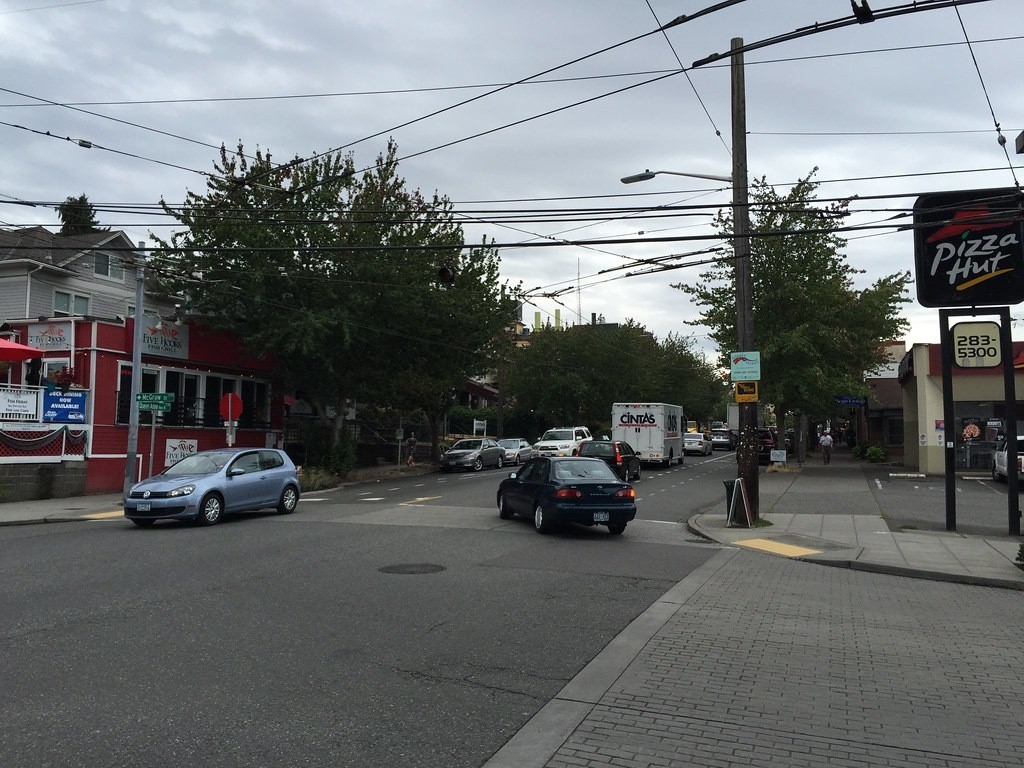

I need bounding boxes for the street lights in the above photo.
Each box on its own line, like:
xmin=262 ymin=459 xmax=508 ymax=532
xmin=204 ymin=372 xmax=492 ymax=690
xmin=620 ymin=37 xmax=760 ymax=527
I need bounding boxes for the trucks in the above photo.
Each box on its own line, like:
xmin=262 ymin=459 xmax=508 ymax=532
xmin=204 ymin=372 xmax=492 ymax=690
xmin=611 ymin=403 xmax=688 ymax=467
xmin=726 ymin=403 xmax=765 ymax=441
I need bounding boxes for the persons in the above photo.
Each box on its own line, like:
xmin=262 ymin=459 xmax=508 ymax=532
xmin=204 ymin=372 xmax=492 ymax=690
xmin=819 ymin=430 xmax=834 ymax=466
xmin=405 ymin=432 xmax=418 ymax=466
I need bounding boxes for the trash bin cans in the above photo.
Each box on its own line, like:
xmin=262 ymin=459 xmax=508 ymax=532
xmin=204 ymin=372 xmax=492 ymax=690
xmin=722 ymin=479 xmax=736 ymax=521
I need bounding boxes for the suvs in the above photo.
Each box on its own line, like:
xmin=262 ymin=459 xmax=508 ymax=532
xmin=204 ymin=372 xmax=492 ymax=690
xmin=992 ymin=436 xmax=1024 ymax=482
xmin=758 ymin=426 xmax=778 ymax=466
xmin=530 ymin=426 xmax=594 ymax=459
xmin=708 ymin=429 xmax=736 ymax=451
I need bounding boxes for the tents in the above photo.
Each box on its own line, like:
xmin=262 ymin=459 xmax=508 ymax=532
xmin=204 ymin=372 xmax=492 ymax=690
xmin=0 ymin=337 xmax=46 ymax=386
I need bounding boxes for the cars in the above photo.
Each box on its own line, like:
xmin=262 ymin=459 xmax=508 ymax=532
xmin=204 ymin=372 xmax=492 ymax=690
xmin=682 ymin=432 xmax=712 ymax=456
xmin=439 ymin=438 xmax=506 ymax=471
xmin=496 ymin=457 xmax=637 ymax=535
xmin=575 ymin=435 xmax=642 ymax=482
xmin=496 ymin=438 xmax=532 ymax=465
xmin=687 ymin=421 xmax=698 ymax=433
xmin=123 ymin=447 xmax=302 ymax=525
xmin=770 ymin=426 xmax=794 ymax=453
